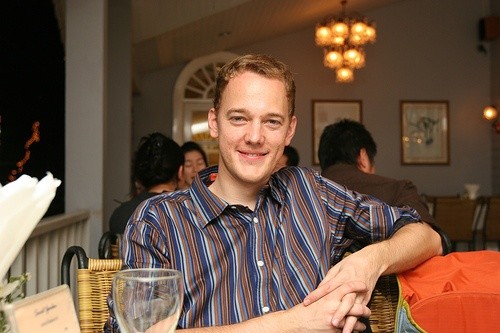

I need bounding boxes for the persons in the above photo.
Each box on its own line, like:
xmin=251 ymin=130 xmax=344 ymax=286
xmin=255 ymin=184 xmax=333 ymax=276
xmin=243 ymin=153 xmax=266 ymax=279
xmin=102 ymin=53 xmax=456 ymax=333
xmin=108 ymin=119 xmax=450 ymax=252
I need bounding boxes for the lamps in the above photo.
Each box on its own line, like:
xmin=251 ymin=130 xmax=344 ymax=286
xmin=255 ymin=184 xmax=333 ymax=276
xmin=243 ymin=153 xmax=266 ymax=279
xmin=484 ymin=105 xmax=500 ymax=134
xmin=314 ymin=1 xmax=377 ymax=86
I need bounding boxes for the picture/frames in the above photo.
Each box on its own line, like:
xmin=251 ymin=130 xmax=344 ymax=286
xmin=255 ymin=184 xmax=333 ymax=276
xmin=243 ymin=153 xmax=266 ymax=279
xmin=311 ymin=99 xmax=365 ymax=165
xmin=399 ymin=99 xmax=451 ymax=166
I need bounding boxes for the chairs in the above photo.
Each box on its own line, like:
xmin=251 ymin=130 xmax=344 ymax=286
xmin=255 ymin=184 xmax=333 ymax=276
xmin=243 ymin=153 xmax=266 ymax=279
xmin=336 ymin=244 xmax=397 ymax=333
xmin=449 ymin=195 xmax=491 ymax=251
xmin=59 ymin=230 xmax=123 ymax=332
xmin=394 ymin=250 xmax=500 ymax=333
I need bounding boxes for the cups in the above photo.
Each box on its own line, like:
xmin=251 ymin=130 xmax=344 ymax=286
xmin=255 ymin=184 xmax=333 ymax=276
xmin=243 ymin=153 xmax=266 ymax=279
xmin=113 ymin=268 xmax=184 ymax=333
